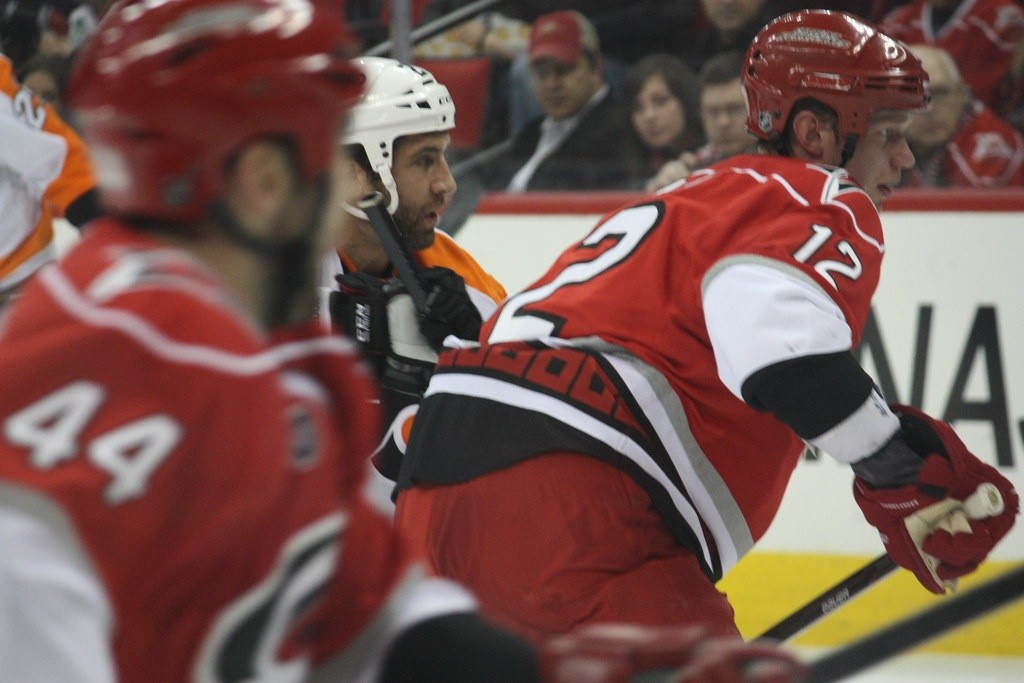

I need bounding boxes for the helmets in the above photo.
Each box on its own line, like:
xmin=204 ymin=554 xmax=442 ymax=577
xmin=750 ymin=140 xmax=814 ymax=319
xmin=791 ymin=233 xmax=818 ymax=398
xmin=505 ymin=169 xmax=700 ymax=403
xmin=340 ymin=57 xmax=455 ymax=217
xmin=66 ymin=1 xmax=372 ymax=224
xmin=738 ymin=8 xmax=933 ymax=145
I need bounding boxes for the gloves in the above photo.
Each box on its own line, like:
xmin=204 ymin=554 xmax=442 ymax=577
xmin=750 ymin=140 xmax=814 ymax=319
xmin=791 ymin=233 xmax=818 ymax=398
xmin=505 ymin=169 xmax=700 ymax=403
xmin=329 ymin=266 xmax=481 ymax=373
xmin=851 ymin=399 xmax=1019 ymax=594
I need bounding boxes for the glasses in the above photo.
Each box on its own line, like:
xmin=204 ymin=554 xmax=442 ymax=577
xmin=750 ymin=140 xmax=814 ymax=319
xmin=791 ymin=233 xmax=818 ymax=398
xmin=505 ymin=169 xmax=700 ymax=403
xmin=932 ymin=81 xmax=958 ymax=99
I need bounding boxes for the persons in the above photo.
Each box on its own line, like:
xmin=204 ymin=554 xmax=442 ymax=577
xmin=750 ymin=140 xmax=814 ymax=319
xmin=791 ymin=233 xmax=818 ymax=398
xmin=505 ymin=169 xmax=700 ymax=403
xmin=28 ymin=0 xmax=98 ymax=76
xmin=0 ymin=2 xmax=810 ymax=683
xmin=389 ymin=10 xmax=1020 ymax=683
xmin=445 ymin=10 xmax=655 ymax=192
xmin=893 ymin=43 xmax=1024 ymax=191
xmin=0 ymin=53 xmax=104 ymax=324
xmin=643 ymin=50 xmax=759 ymax=192
xmin=691 ymin=0 xmax=782 ymax=69
xmin=621 ymin=53 xmax=706 ymax=189
xmin=874 ymin=0 xmax=1024 ymax=118
xmin=312 ymin=52 xmax=510 ymax=523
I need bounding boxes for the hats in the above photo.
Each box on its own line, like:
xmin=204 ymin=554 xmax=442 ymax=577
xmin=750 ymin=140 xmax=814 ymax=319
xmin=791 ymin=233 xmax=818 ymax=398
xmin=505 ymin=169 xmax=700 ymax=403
xmin=526 ymin=9 xmax=599 ymax=68
